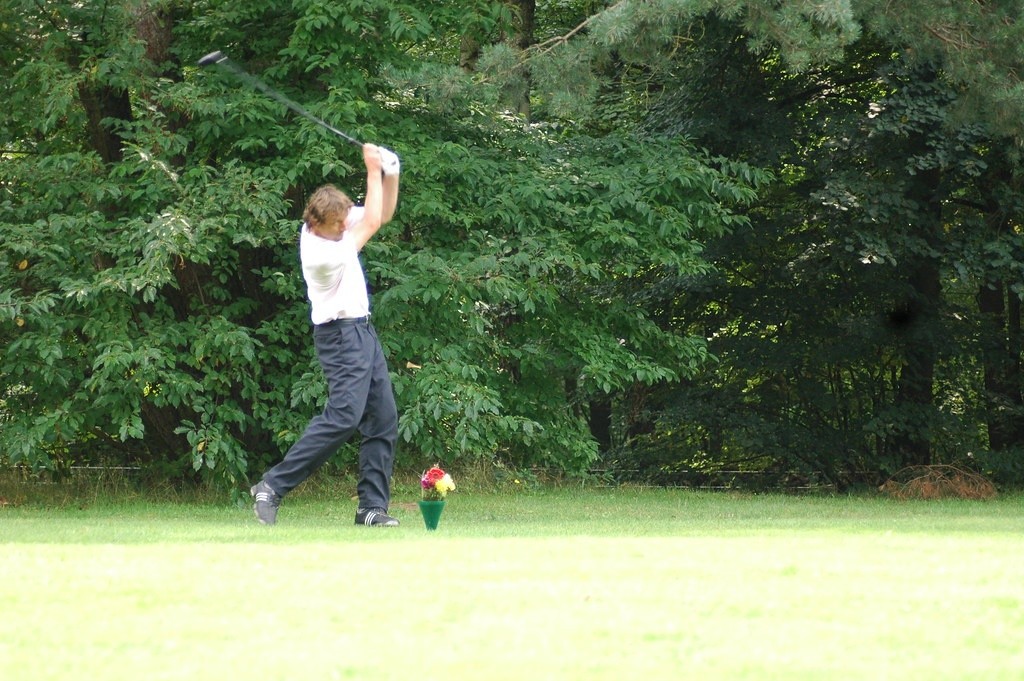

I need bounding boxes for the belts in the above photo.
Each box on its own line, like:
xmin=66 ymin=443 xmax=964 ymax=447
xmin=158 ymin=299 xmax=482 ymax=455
xmin=322 ymin=316 xmax=370 ymax=325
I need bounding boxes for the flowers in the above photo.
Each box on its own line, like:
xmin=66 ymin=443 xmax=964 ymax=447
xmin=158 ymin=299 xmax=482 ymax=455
xmin=421 ymin=465 xmax=456 ymax=502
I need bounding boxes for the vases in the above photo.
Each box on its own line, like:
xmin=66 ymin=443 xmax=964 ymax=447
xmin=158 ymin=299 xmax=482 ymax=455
xmin=417 ymin=500 xmax=446 ymax=531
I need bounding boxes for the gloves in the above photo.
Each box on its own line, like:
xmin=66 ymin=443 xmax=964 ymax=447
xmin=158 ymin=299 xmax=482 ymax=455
xmin=379 ymin=146 xmax=400 ymax=175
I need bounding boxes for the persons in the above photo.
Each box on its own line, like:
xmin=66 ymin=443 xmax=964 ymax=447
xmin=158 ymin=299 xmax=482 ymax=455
xmin=250 ymin=143 xmax=402 ymax=527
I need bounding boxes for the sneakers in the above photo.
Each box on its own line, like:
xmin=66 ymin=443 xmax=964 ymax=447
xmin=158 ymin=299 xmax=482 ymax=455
xmin=250 ymin=480 xmax=281 ymax=527
xmin=355 ymin=507 xmax=400 ymax=527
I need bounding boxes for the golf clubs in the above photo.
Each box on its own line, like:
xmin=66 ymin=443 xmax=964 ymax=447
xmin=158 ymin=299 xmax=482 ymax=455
xmin=197 ymin=50 xmax=397 ymax=166
